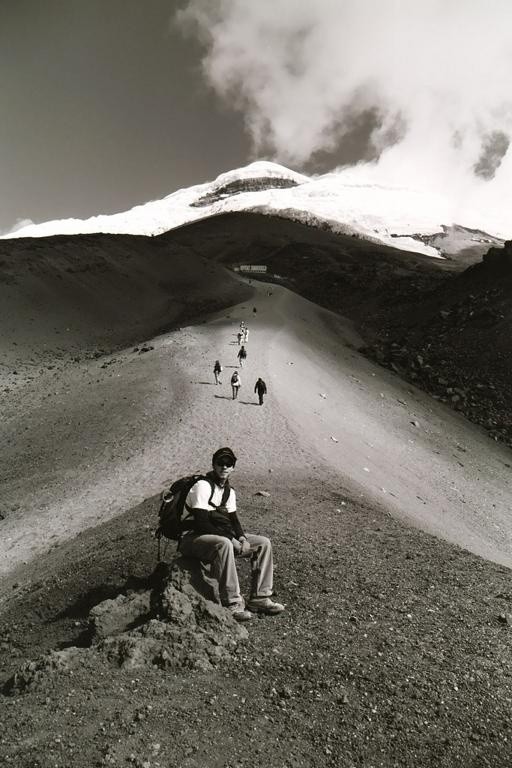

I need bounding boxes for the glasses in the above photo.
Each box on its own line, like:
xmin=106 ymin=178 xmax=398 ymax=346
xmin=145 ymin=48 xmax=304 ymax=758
xmin=215 ymin=460 xmax=234 ymax=468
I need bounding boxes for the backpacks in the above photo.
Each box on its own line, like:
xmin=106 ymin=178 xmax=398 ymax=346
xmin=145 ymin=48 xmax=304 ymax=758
xmin=155 ymin=473 xmax=231 ymax=540
xmin=232 ymin=375 xmax=238 ymax=383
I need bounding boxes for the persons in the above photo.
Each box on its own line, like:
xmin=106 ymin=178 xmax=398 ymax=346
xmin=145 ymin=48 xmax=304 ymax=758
xmin=213 ymin=307 xmax=267 ymax=405
xmin=177 ymin=447 xmax=285 ymax=620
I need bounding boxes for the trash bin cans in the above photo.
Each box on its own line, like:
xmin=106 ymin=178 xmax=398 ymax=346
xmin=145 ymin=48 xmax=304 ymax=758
xmin=249 ymin=280 xmax=251 ymax=284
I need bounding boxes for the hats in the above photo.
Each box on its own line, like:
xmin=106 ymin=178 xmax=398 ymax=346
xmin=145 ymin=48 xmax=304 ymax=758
xmin=212 ymin=447 xmax=237 ymax=463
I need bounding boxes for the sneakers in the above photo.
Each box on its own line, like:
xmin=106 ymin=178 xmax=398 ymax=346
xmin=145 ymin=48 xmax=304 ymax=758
xmin=227 ymin=603 xmax=251 ymax=621
xmin=248 ymin=596 xmax=285 ymax=612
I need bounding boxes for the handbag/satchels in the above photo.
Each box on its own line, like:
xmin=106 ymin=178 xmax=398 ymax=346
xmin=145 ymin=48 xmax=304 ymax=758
xmin=199 ymin=509 xmax=234 ymax=538
